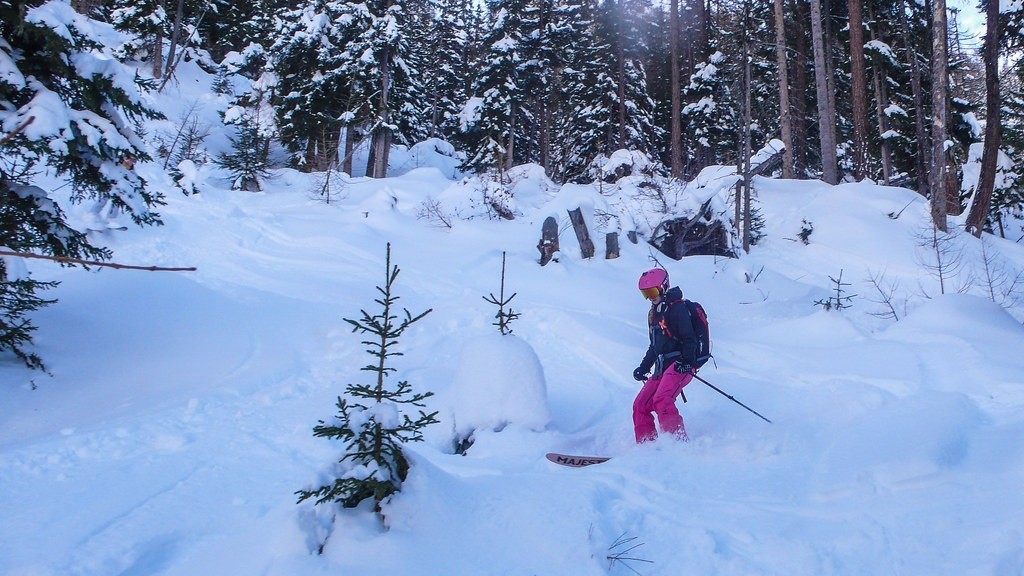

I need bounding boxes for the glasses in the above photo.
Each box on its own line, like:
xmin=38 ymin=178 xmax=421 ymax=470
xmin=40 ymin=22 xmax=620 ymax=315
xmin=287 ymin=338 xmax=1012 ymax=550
xmin=640 ymin=287 xmax=659 ymax=300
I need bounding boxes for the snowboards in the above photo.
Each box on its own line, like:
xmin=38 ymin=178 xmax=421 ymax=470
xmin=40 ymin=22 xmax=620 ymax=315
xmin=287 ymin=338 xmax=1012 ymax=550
xmin=546 ymin=452 xmax=612 ymax=468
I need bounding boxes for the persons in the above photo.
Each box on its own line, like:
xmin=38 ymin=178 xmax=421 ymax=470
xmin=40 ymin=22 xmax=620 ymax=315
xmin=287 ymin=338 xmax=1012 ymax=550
xmin=632 ymin=268 xmax=699 ymax=444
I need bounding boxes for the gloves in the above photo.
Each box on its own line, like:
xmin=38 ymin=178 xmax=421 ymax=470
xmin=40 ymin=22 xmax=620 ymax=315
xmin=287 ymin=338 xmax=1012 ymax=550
xmin=633 ymin=367 xmax=648 ymax=380
xmin=674 ymin=360 xmax=693 ymax=373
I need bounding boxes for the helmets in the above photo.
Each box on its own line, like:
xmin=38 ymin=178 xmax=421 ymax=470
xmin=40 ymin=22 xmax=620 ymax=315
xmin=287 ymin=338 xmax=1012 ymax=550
xmin=639 ymin=268 xmax=670 ymax=291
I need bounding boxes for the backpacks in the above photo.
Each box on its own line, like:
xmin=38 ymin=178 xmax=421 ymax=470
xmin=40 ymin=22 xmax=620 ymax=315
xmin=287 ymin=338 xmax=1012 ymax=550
xmin=648 ymin=299 xmax=709 ymax=369
